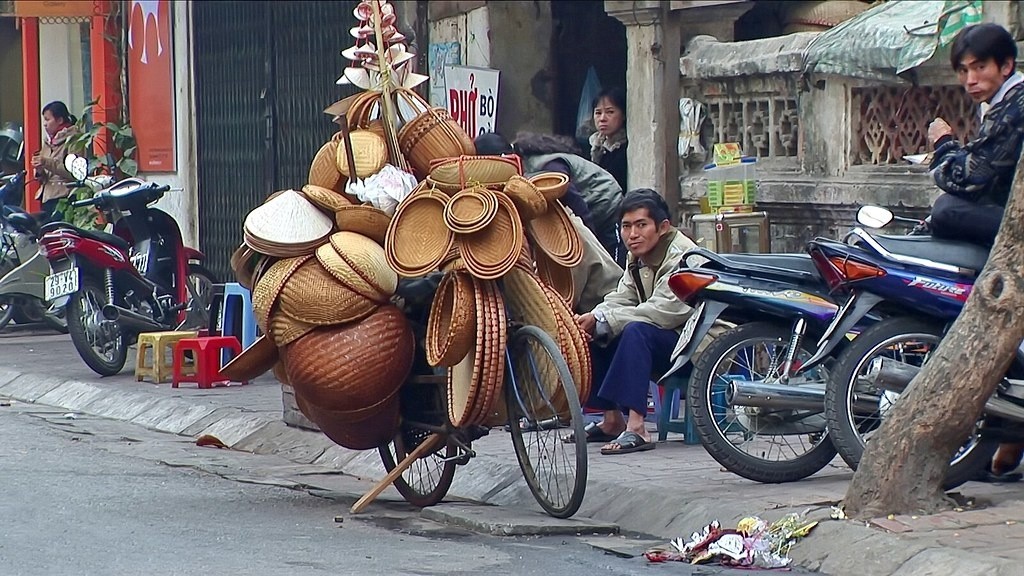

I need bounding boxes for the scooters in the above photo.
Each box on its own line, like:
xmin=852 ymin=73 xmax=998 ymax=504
xmin=0 ymin=159 xmax=70 ymax=335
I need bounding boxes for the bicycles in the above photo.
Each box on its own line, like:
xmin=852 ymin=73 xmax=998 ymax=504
xmin=378 ymin=268 xmax=588 ymax=520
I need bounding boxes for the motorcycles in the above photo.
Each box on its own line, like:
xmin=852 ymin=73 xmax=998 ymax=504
xmin=655 ymin=204 xmax=1024 ymax=493
xmin=36 ymin=153 xmax=223 ymax=377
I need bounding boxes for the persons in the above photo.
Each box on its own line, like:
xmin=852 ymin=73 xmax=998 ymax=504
xmin=588 ymin=85 xmax=628 ymax=198
xmin=563 ymin=187 xmax=737 ymax=455
xmin=928 ymin=21 xmax=1024 ymax=247
xmin=473 ymin=130 xmax=628 ymax=433
xmin=30 ymin=101 xmax=85 ymax=226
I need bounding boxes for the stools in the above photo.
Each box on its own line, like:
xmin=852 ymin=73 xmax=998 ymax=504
xmin=577 ymin=374 xmax=754 ymax=445
xmin=134 ymin=282 xmax=258 ymax=389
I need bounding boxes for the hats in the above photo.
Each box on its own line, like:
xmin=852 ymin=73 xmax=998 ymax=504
xmin=230 ymin=189 xmax=335 ymax=285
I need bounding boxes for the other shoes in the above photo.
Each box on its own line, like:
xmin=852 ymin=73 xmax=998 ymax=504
xmin=990 ymin=441 xmax=1024 ymax=476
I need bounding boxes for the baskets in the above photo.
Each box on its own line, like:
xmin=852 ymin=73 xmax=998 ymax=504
xmin=307 ymin=88 xmax=590 ymax=431
xmin=218 ymin=232 xmax=416 ymax=451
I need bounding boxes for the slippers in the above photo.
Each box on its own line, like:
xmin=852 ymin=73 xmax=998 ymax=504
xmin=560 ymin=421 xmax=619 ymax=442
xmin=505 ymin=414 xmax=568 ymax=430
xmin=601 ymin=431 xmax=656 ymax=455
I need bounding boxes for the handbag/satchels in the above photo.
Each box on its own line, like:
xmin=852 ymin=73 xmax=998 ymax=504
xmin=575 ymin=65 xmax=606 ymax=143
